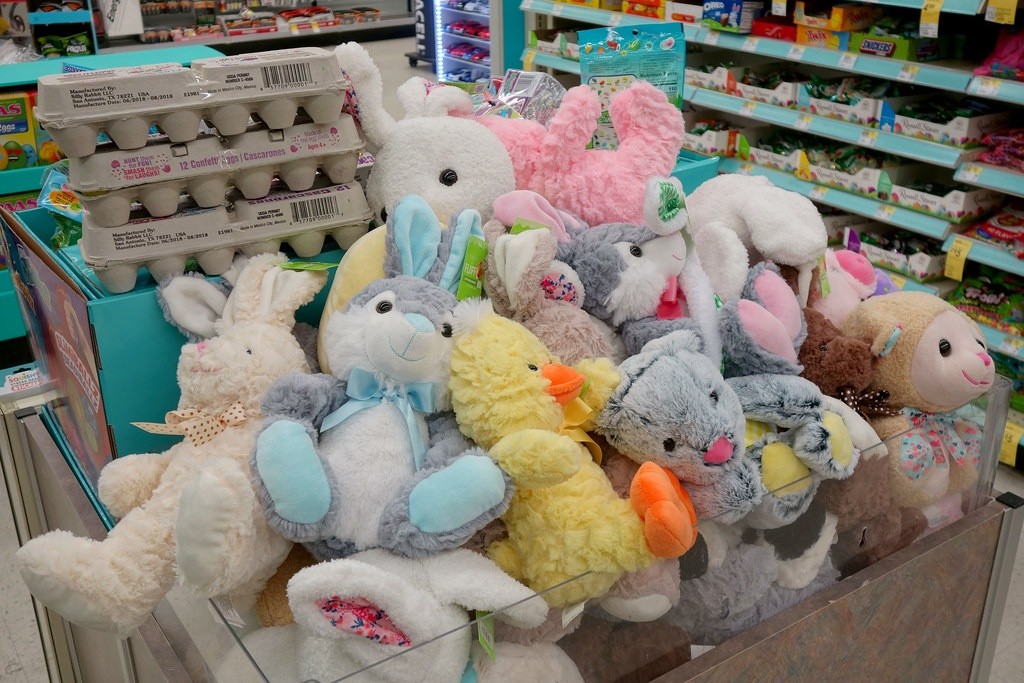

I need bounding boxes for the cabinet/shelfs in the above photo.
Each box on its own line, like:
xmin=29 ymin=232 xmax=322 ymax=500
xmin=439 ymin=6 xmax=492 ymax=82
xmin=518 ymin=0 xmax=1024 ymax=476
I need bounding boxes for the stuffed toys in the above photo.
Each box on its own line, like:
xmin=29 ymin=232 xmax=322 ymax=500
xmin=319 ymin=42 xmax=996 ymax=683
xmin=446 ymin=297 xmax=697 ymax=606
xmin=289 ymin=549 xmax=586 ymax=682
xmin=247 ymin=193 xmax=516 ymax=558
xmin=14 ymin=251 xmax=330 ymax=639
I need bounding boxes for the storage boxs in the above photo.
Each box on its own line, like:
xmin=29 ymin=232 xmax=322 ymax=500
xmin=0 ymin=0 xmax=1002 ymax=472
